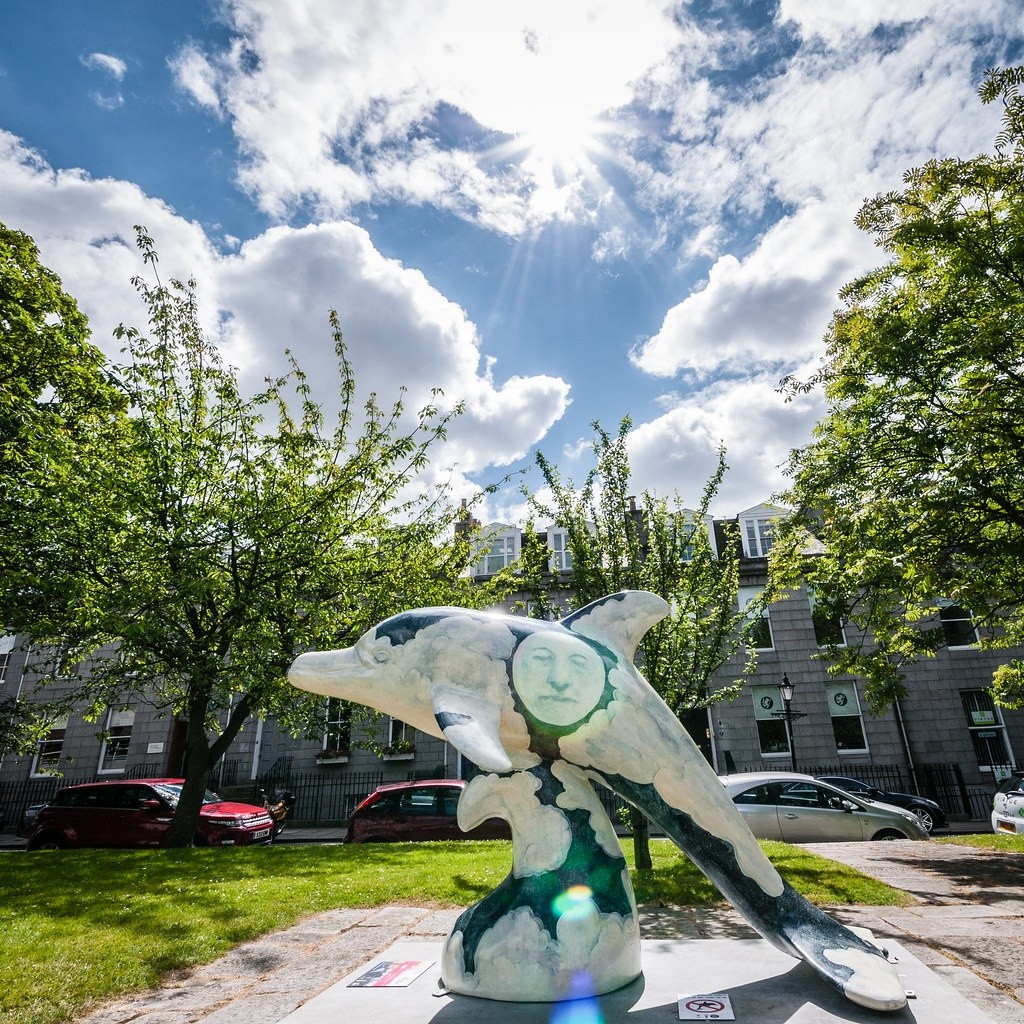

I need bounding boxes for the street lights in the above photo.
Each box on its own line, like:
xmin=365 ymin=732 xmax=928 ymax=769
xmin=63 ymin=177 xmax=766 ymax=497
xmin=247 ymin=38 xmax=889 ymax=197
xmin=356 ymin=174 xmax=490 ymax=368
xmin=772 ymin=670 xmax=811 ymax=774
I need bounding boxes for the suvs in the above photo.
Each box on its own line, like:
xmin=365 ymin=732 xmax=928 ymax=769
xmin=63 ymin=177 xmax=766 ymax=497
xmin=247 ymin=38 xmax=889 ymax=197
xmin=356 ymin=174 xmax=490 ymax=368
xmin=31 ymin=777 xmax=272 ymax=851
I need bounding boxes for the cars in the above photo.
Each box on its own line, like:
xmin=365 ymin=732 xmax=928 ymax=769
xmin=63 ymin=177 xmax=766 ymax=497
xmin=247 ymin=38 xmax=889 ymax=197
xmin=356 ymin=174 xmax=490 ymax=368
xmin=763 ymin=774 xmax=949 ymax=840
xmin=990 ymin=770 xmax=1024 ymax=839
xmin=693 ymin=770 xmax=930 ymax=846
xmin=24 ymin=799 xmax=50 ymax=836
xmin=343 ymin=778 xmax=513 ymax=844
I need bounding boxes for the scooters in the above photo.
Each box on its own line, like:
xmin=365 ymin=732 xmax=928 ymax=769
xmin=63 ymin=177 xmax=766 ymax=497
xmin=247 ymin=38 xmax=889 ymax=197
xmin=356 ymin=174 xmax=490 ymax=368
xmin=256 ymin=788 xmax=297 ymax=834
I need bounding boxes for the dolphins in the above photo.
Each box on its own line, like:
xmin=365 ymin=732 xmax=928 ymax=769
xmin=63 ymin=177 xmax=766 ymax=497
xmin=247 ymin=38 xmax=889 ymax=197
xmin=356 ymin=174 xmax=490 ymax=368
xmin=283 ymin=589 xmax=910 ymax=1012
